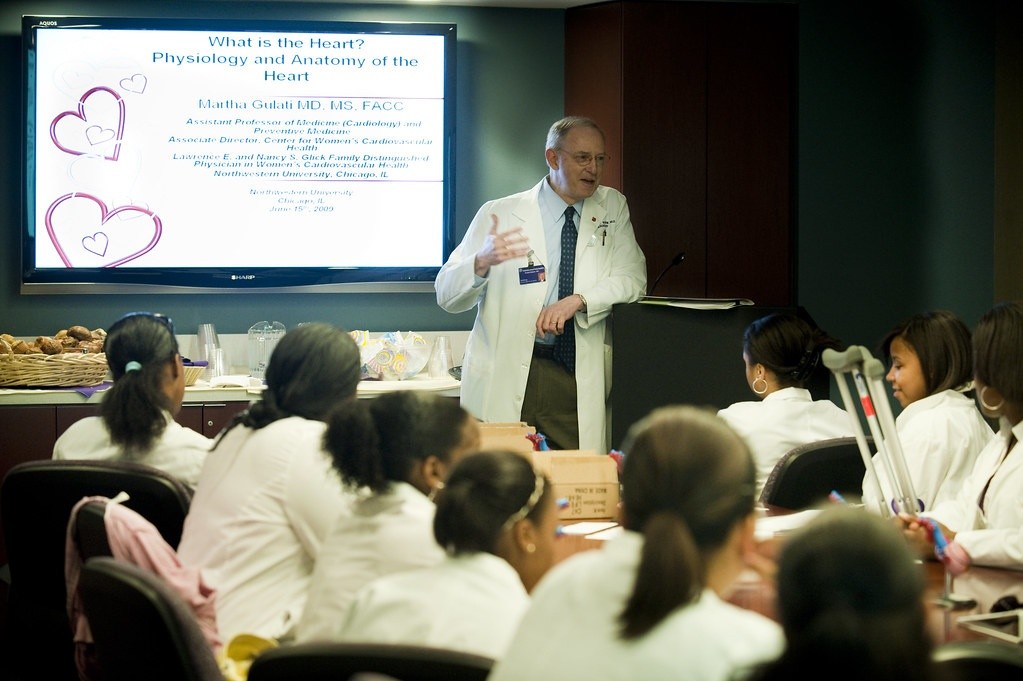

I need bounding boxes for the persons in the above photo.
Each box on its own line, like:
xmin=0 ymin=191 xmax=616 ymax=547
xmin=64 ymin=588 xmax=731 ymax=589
xmin=52 ymin=311 xmax=212 ymax=491
xmin=539 ymin=273 xmax=545 ymax=282
xmin=171 ymin=305 xmax=1023 ymax=681
xmin=431 ymin=115 xmax=648 ymax=449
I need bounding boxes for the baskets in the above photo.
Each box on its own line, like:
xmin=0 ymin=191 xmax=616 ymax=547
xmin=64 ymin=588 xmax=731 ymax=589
xmin=183 ymin=366 xmax=203 ymax=386
xmin=0 ymin=352 xmax=110 ymax=387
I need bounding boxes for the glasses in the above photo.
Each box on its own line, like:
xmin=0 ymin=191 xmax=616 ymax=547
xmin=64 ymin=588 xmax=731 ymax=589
xmin=559 ymin=147 xmax=611 ymax=168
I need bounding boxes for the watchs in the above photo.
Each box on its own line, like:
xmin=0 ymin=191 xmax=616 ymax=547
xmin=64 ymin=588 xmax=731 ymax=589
xmin=575 ymin=294 xmax=588 ymax=312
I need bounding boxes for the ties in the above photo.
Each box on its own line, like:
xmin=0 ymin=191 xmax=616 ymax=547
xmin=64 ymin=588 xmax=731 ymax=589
xmin=553 ymin=206 xmax=578 ymax=376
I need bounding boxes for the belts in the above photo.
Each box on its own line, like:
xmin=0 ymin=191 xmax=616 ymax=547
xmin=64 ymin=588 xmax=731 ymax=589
xmin=534 ymin=342 xmax=555 ymax=360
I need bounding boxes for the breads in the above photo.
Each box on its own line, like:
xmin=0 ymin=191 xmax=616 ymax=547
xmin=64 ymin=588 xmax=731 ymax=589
xmin=0 ymin=325 xmax=107 ymax=354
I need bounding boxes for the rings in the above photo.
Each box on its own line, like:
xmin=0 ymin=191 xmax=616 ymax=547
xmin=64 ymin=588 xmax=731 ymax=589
xmin=551 ymin=321 xmax=557 ymax=323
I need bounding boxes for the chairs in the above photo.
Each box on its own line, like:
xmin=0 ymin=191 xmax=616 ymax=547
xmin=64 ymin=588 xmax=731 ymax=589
xmin=0 ymin=437 xmax=1023 ymax=681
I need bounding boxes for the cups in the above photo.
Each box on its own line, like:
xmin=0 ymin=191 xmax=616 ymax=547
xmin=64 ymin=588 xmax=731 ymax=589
xmin=199 ymin=323 xmax=222 ymax=386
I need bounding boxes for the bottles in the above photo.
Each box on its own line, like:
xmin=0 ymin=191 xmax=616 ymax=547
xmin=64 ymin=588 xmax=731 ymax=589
xmin=429 ymin=337 xmax=448 ymax=379
